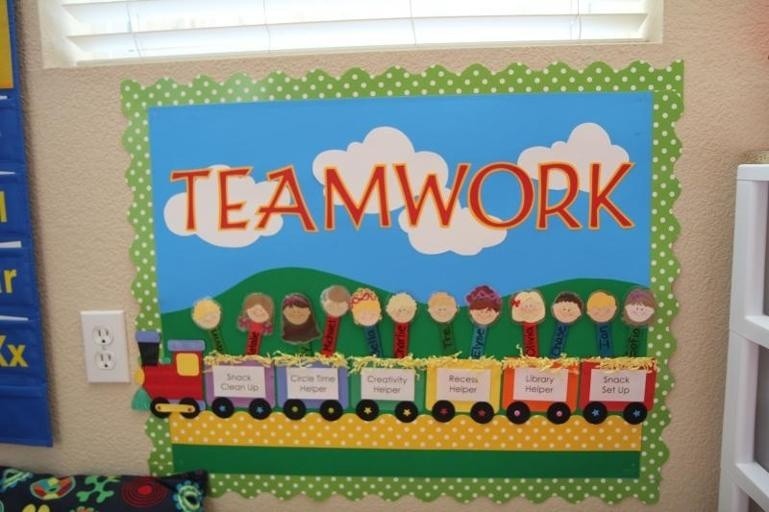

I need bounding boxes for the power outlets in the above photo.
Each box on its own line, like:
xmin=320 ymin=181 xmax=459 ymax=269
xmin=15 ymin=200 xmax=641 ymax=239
xmin=81 ymin=309 xmax=130 ymax=383
